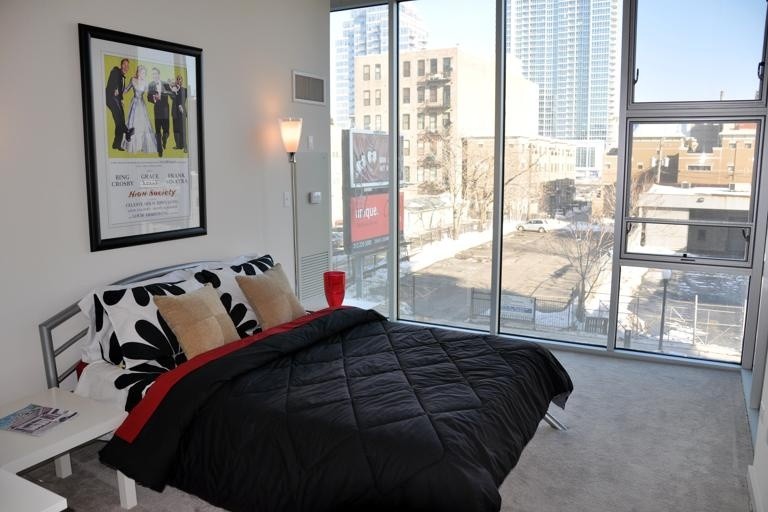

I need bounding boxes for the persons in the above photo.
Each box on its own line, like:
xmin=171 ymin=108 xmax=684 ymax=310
xmin=105 ymin=58 xmax=135 ymax=151
xmin=168 ymin=74 xmax=188 ymax=153
xmin=121 ymin=65 xmax=162 ymax=154
xmin=147 ymin=67 xmax=176 ymax=157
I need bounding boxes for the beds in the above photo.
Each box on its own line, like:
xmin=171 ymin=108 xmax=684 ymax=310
xmin=37 ymin=261 xmax=574 ymax=512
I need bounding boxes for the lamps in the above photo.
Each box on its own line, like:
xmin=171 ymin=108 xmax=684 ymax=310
xmin=279 ymin=117 xmax=303 ymax=300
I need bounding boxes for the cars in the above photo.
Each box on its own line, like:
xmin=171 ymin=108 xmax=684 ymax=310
xmin=331 ymin=225 xmax=343 ymax=250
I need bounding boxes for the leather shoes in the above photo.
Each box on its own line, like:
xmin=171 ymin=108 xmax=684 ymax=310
xmin=112 ymin=129 xmax=188 ymax=158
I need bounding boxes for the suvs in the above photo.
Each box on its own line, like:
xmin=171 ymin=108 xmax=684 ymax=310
xmin=514 ymin=217 xmax=551 ymax=234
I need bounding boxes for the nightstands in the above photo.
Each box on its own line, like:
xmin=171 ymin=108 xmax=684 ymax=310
xmin=306 ymin=299 xmax=380 ymax=311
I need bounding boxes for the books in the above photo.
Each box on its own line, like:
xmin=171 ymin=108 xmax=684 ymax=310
xmin=0 ymin=400 xmax=77 ymax=441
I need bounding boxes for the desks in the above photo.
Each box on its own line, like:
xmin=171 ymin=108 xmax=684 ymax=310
xmin=0 ymin=390 xmax=137 ymax=512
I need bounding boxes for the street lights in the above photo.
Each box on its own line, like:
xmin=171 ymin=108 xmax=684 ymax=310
xmin=657 ymin=268 xmax=671 ymax=351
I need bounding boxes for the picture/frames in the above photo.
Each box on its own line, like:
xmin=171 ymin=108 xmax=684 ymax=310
xmin=291 ymin=70 xmax=326 ymax=106
xmin=77 ymin=23 xmax=207 ymax=253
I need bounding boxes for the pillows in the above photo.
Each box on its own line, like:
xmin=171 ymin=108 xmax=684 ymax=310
xmin=154 ymin=282 xmax=239 ymax=362
xmin=194 ymin=254 xmax=262 ymax=339
xmin=78 ymin=271 xmax=193 ymax=363
xmin=234 ymin=262 xmax=307 ymax=331
xmin=189 ymin=256 xmax=257 ymax=272
xmin=97 ymin=279 xmax=205 ymax=373
